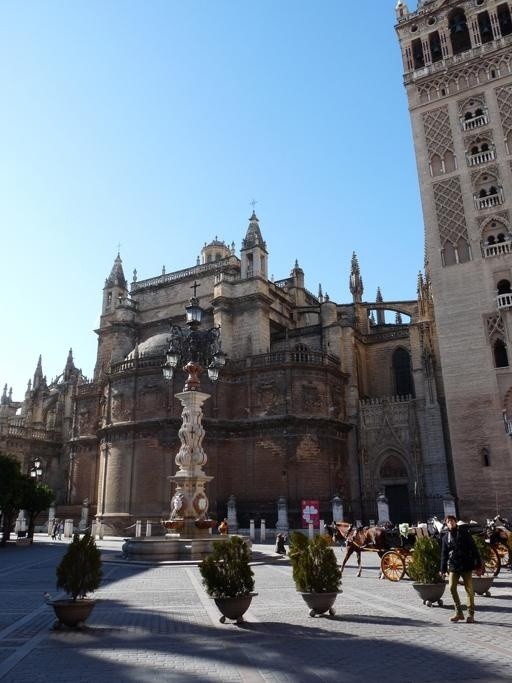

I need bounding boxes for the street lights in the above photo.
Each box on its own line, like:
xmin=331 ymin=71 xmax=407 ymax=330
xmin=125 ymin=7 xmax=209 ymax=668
xmin=160 ymin=281 xmax=227 ymax=539
xmin=28 ymin=455 xmax=43 ymax=485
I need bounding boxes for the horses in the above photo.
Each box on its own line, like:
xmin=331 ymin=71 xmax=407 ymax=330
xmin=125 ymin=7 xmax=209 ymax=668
xmin=324 ymin=520 xmax=389 ymax=580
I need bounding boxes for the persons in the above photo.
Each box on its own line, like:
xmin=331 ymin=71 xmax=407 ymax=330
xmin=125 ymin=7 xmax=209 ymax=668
xmin=276 ymin=533 xmax=288 ymax=556
xmin=439 ymin=515 xmax=482 ymax=623
xmin=221 ymin=518 xmax=228 ymax=535
xmin=50 ymin=519 xmax=63 ymax=540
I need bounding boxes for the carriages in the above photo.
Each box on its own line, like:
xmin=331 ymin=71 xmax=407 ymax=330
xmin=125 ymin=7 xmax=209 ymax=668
xmin=332 ymin=515 xmax=512 ymax=582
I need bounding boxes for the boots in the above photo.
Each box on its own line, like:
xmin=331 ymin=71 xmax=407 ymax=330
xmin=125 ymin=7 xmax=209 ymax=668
xmin=449 ymin=613 xmax=465 ymax=622
xmin=467 ymin=612 xmax=474 ymax=623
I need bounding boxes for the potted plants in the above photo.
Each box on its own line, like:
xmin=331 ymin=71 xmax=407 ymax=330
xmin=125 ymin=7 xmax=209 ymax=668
xmin=199 ymin=529 xmax=343 ymax=623
xmin=407 ymin=534 xmax=494 ymax=606
xmin=46 ymin=531 xmax=109 ymax=631
